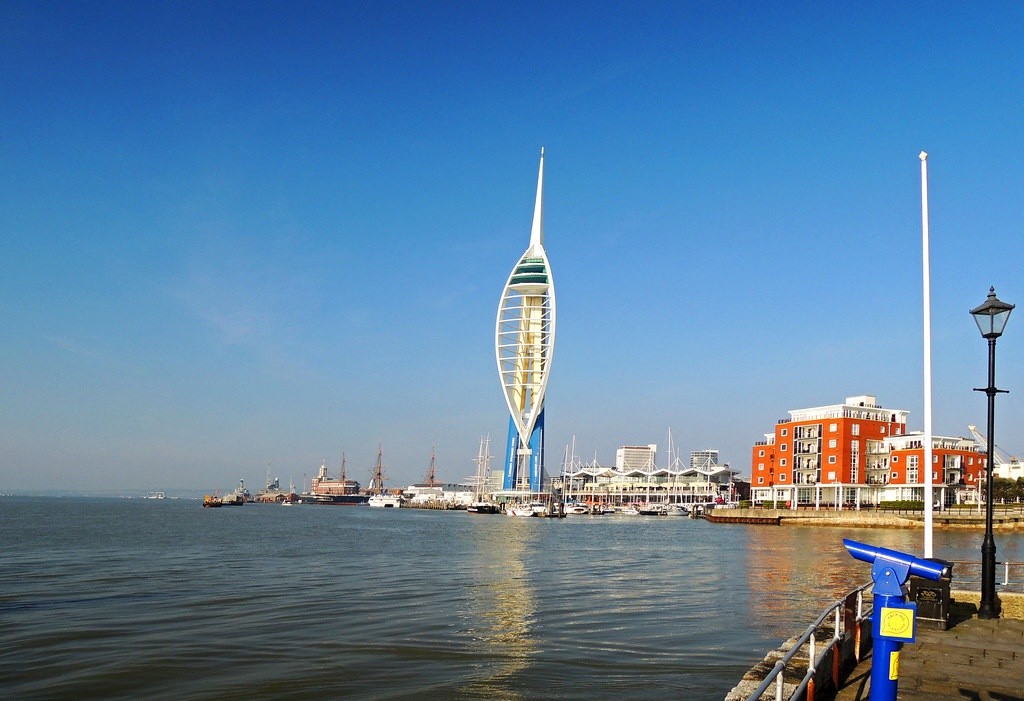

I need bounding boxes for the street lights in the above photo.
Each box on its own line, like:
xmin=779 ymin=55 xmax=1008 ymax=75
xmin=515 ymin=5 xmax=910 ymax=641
xmin=969 ymin=285 xmax=1015 ymax=619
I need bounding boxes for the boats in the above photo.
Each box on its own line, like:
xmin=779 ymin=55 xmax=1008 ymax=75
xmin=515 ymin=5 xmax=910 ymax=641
xmin=281 ymin=500 xmax=292 ymax=507
xmin=203 ymin=495 xmax=222 ymax=507
xmin=222 ymin=494 xmax=244 ymax=506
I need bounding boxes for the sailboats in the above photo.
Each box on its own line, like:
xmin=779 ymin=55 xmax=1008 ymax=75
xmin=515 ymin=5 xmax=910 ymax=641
xmin=468 ymin=426 xmax=714 ymax=518
